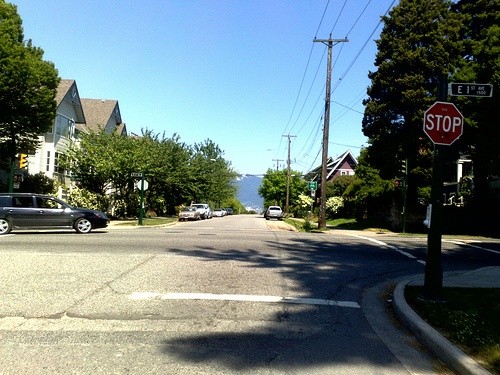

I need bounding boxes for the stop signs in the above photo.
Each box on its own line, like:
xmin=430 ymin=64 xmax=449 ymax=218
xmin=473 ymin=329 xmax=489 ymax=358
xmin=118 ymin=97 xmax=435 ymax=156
xmin=423 ymin=102 xmax=464 ymax=145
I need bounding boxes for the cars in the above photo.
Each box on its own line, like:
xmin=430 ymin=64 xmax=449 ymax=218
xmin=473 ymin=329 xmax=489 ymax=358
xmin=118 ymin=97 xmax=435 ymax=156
xmin=191 ymin=204 xmax=212 ymax=219
xmin=212 ymin=208 xmax=233 ymax=217
xmin=264 ymin=206 xmax=283 ymax=220
xmin=0 ymin=193 xmax=110 ymax=235
xmin=179 ymin=207 xmax=199 ymax=221
